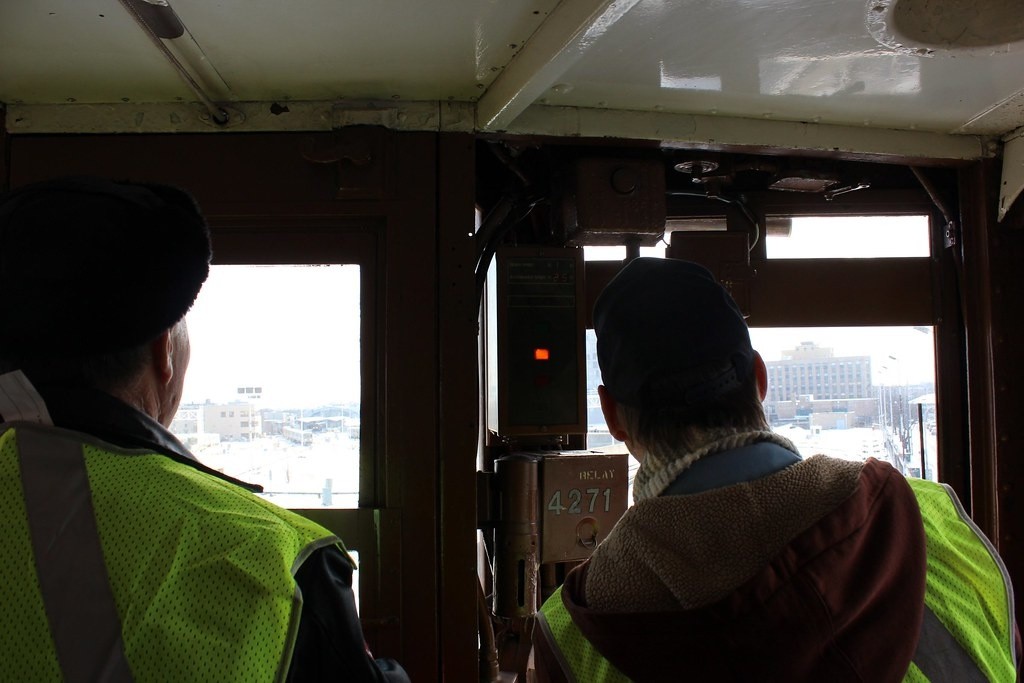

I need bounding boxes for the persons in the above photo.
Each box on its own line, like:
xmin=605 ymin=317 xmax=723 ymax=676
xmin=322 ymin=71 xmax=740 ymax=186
xmin=0 ymin=179 xmax=407 ymax=683
xmin=535 ymin=257 xmax=1024 ymax=683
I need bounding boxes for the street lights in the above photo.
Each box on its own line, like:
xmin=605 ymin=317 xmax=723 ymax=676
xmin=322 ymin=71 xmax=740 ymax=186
xmin=888 ymin=355 xmax=912 ymax=456
xmin=882 ymin=365 xmax=894 ymax=437
